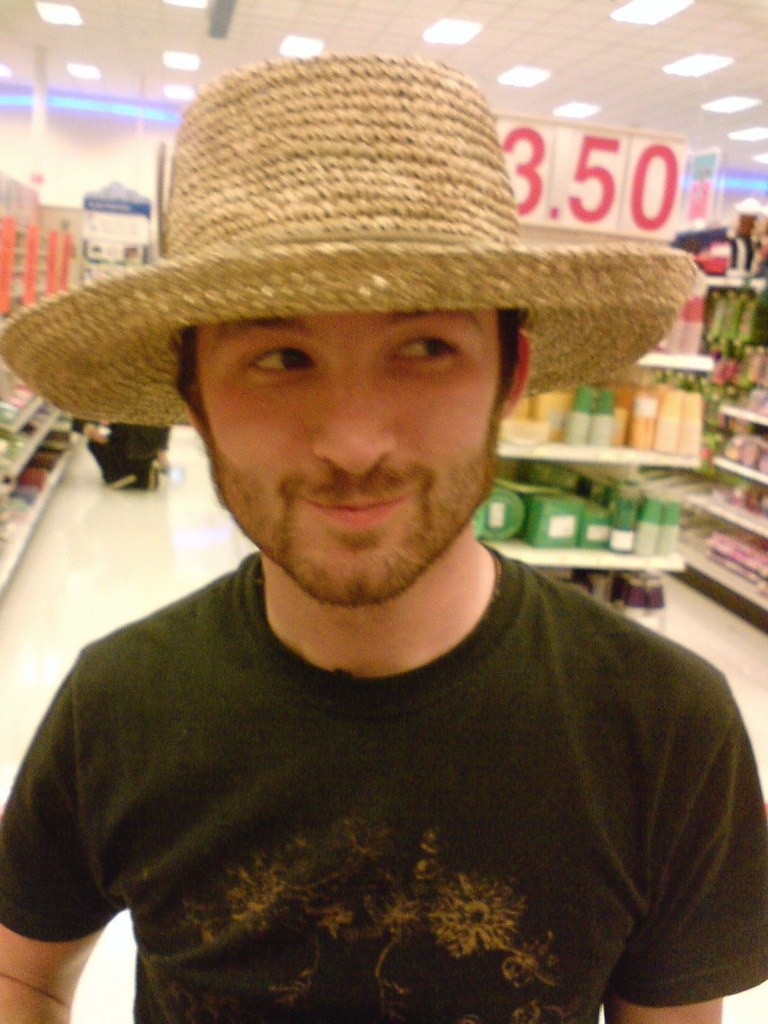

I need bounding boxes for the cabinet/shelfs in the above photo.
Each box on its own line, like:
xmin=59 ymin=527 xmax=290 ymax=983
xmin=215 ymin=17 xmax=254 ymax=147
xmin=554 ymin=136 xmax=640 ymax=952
xmin=652 ymin=276 xmax=768 ymax=632
xmin=490 ymin=440 xmax=701 ymax=573
xmin=0 ymin=175 xmax=77 ymax=602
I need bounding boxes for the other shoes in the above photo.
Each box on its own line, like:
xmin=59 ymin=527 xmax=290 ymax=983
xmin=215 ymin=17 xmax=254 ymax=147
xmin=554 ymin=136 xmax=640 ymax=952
xmin=110 ymin=473 xmax=137 ymax=489
xmin=139 ymin=460 xmax=159 ymax=490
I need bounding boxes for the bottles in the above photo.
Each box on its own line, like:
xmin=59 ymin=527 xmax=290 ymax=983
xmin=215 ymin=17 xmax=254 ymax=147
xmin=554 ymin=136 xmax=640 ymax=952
xmin=561 ymin=377 xmax=703 ymax=556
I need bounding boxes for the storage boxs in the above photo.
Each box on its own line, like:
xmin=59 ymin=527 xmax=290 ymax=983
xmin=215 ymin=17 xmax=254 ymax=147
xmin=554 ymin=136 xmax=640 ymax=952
xmin=513 ymin=485 xmax=610 ymax=550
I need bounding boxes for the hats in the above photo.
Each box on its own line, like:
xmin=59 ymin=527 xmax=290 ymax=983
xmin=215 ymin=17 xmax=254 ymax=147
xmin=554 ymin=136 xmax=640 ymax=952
xmin=0 ymin=51 xmax=697 ymax=425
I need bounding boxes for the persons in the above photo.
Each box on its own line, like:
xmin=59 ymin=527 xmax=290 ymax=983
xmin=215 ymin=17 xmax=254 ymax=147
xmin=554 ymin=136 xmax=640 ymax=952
xmin=87 ymin=419 xmax=171 ymax=490
xmin=0 ymin=55 xmax=767 ymax=1024
